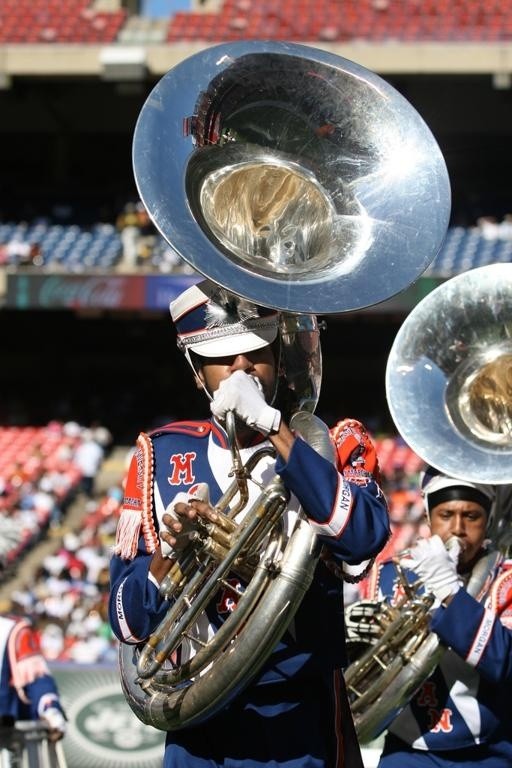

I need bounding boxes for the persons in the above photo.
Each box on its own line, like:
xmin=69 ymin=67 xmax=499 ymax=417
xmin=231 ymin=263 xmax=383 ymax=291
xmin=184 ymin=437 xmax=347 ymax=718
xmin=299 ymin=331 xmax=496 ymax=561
xmin=0 ymin=199 xmax=512 ymax=768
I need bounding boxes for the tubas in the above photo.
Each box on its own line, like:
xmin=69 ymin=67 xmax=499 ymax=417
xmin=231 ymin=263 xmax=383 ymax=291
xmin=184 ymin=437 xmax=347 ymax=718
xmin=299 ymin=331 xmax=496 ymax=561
xmin=119 ymin=40 xmax=452 ymax=730
xmin=344 ymin=262 xmax=511 ymax=742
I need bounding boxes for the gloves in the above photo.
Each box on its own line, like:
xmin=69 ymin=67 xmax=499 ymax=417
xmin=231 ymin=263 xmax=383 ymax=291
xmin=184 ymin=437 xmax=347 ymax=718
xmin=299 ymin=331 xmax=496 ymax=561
xmin=399 ymin=536 xmax=466 ymax=603
xmin=210 ymin=370 xmax=282 ymax=439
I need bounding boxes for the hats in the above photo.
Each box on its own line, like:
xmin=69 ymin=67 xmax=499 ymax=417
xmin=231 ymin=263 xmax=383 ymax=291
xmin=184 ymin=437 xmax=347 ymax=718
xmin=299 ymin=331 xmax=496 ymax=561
xmin=169 ymin=278 xmax=282 ymax=359
xmin=419 ymin=464 xmax=499 ymax=516
